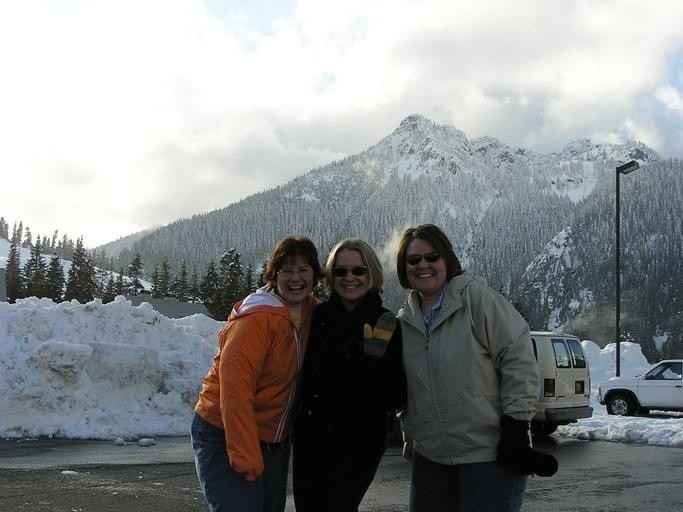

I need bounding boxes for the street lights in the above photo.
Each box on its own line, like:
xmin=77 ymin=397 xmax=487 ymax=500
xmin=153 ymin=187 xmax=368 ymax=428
xmin=613 ymin=160 xmax=640 ymax=377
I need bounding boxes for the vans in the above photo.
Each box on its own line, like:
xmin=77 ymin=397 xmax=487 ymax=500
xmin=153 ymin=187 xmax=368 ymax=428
xmin=527 ymin=329 xmax=594 ymax=436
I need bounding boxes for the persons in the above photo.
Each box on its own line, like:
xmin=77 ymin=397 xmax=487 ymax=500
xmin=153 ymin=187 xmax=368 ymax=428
xmin=290 ymin=236 xmax=410 ymax=512
xmin=395 ymin=223 xmax=543 ymax=512
xmin=188 ymin=233 xmax=325 ymax=512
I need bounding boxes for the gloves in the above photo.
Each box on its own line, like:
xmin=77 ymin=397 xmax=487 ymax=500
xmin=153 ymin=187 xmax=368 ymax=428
xmin=496 ymin=414 xmax=530 ymax=477
xmin=362 ymin=311 xmax=397 ymax=358
xmin=495 ymin=441 xmax=559 ymax=477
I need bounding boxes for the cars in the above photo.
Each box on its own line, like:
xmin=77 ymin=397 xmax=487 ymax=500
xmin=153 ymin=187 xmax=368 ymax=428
xmin=595 ymin=358 xmax=682 ymax=417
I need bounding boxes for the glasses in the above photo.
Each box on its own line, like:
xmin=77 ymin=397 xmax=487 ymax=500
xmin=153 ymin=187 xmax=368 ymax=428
xmin=333 ymin=265 xmax=369 ymax=277
xmin=276 ymin=265 xmax=314 ymax=278
xmin=405 ymin=251 xmax=441 ymax=265
